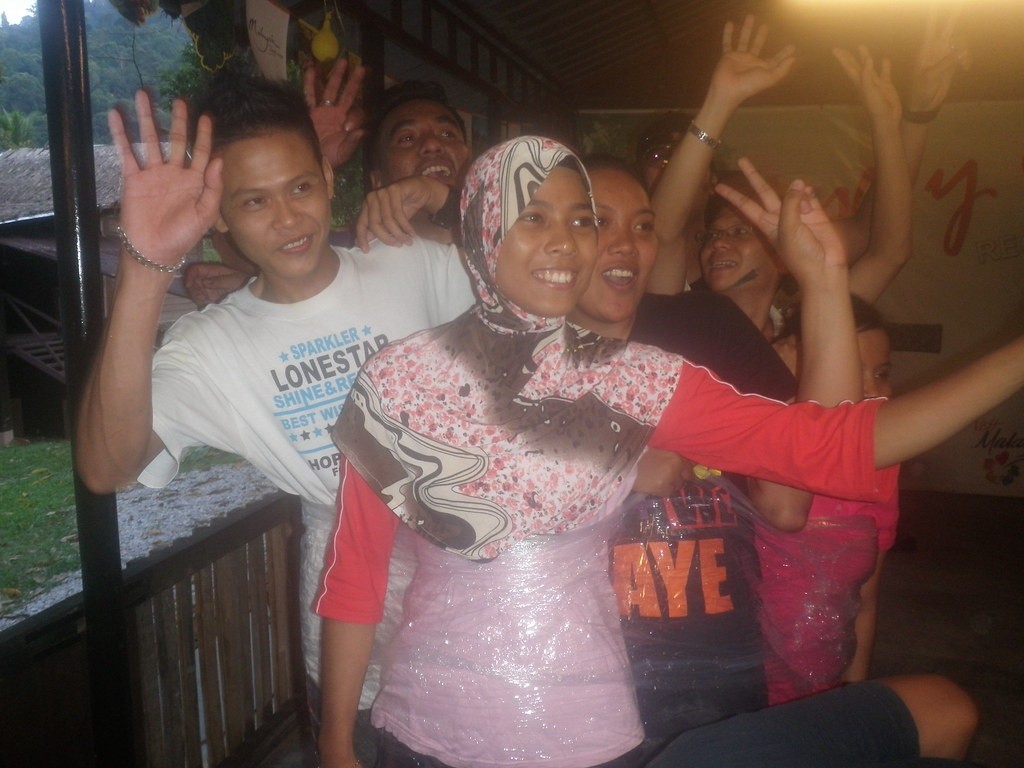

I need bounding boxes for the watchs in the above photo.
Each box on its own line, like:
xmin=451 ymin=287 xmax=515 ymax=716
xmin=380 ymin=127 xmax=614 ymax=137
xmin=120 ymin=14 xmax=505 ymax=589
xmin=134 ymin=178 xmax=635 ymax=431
xmin=687 ymin=120 xmax=722 ymax=151
xmin=428 ymin=183 xmax=460 ymax=231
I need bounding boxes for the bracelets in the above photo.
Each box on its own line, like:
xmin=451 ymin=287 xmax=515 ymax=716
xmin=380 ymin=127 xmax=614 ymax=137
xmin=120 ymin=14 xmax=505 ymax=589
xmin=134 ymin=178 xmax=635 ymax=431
xmin=116 ymin=220 xmax=188 ymax=276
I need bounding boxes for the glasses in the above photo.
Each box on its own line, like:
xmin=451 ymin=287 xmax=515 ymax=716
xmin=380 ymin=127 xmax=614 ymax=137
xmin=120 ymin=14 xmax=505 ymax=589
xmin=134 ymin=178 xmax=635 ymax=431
xmin=695 ymin=223 xmax=760 ymax=244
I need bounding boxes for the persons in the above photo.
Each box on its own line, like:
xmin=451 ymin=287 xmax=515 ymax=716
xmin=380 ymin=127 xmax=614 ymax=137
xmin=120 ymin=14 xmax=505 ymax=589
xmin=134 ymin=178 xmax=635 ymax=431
xmin=296 ymin=11 xmax=1023 ymax=767
xmin=71 ymin=78 xmax=478 ymax=768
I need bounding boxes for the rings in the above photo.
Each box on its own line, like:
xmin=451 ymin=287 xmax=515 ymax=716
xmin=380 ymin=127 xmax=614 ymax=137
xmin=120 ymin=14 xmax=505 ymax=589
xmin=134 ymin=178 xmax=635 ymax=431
xmin=319 ymin=99 xmax=336 ymax=105
